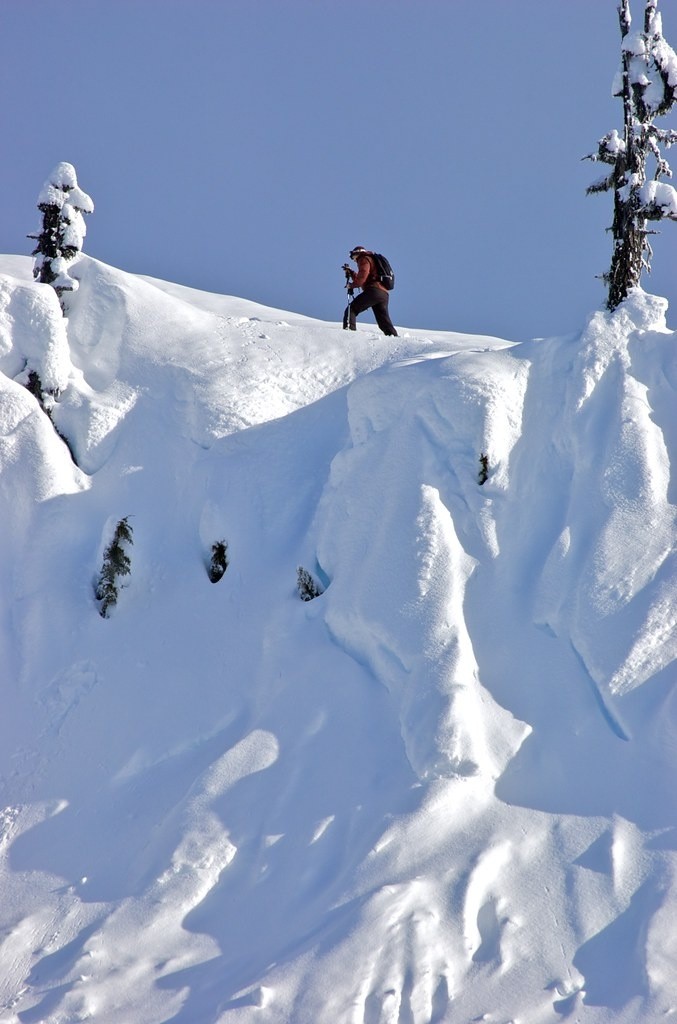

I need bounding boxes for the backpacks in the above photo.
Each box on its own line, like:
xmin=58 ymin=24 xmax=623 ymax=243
xmin=363 ymin=252 xmax=394 ymax=290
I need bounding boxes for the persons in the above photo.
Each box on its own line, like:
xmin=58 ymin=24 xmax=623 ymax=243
xmin=343 ymin=246 xmax=399 ymax=337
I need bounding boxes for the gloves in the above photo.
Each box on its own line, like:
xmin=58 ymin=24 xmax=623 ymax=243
xmin=342 ymin=266 xmax=353 ymax=276
xmin=344 ymin=281 xmax=351 ymax=289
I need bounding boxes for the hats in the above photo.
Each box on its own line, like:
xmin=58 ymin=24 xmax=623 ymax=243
xmin=349 ymin=246 xmax=365 ymax=260
